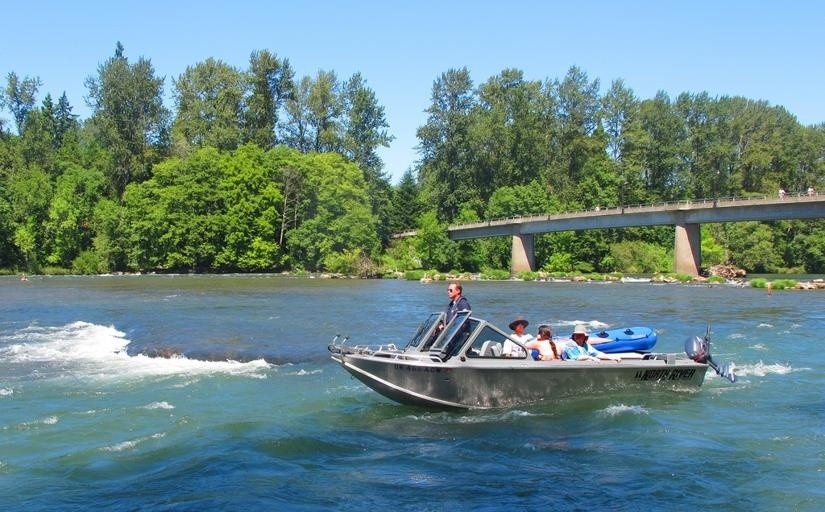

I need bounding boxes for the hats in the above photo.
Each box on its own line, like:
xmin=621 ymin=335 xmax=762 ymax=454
xmin=508 ymin=316 xmax=529 ymax=330
xmin=570 ymin=325 xmax=589 ymax=342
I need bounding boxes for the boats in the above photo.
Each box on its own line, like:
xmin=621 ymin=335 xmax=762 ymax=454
xmin=327 ymin=310 xmax=741 ymax=411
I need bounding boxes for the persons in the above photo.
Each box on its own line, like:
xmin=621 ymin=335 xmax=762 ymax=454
xmin=809 ymin=186 xmax=815 ymax=196
xmin=524 ymin=324 xmax=562 ymax=360
xmin=778 ymin=187 xmax=786 ymax=197
xmin=438 ymin=281 xmax=471 ymax=357
xmin=558 ymin=324 xmax=621 ymax=361
xmin=502 ymin=314 xmax=533 ymax=358
